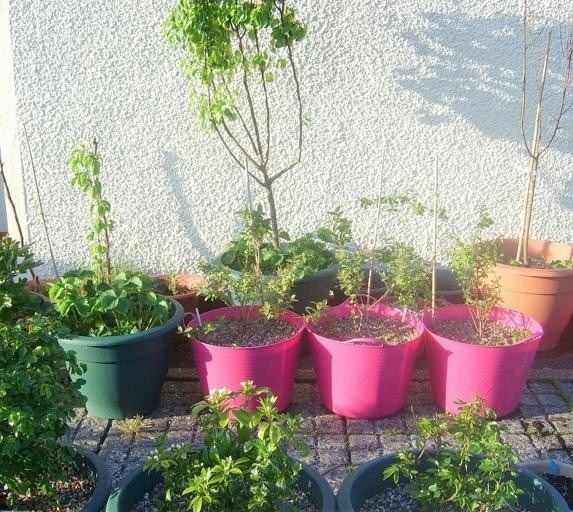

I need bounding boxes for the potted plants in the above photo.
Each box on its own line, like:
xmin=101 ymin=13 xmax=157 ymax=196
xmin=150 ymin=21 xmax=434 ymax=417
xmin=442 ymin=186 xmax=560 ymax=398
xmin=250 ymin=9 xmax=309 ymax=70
xmin=37 ymin=137 xmax=185 ymax=421
xmin=148 ymin=270 xmax=207 ymax=324
xmin=0 ymin=236 xmax=112 ymax=512
xmin=176 ymin=197 xmax=307 ymax=421
xmin=106 ymin=379 xmax=336 ymax=512
xmin=337 ymin=396 xmax=572 ymax=512
xmin=464 ymin=1 xmax=573 ymax=353
xmin=424 ymin=199 xmax=544 ymax=418
xmin=164 ymin=0 xmax=340 ymax=356
xmin=307 ymin=188 xmax=426 ymax=420
